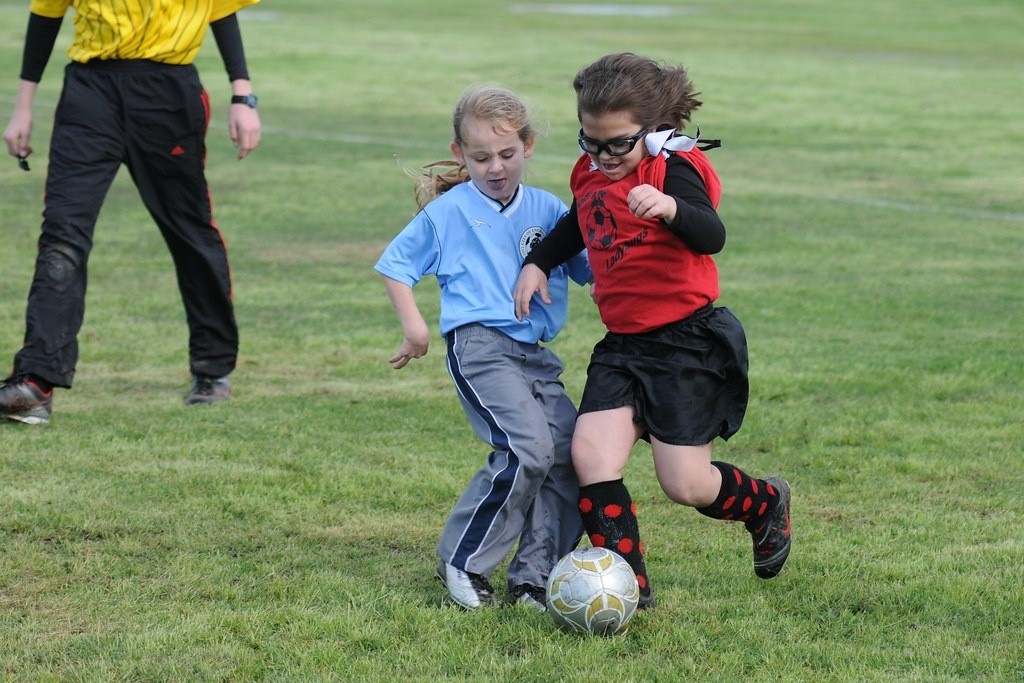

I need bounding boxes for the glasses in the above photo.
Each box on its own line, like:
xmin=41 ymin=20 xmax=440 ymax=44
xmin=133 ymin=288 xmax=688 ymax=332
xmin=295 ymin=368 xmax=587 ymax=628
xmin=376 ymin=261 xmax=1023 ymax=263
xmin=576 ymin=129 xmax=647 ymax=157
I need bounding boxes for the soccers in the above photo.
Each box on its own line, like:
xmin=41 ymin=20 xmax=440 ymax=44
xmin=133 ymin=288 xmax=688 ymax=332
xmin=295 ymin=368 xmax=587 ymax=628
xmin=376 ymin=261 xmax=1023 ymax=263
xmin=544 ymin=545 xmax=641 ymax=635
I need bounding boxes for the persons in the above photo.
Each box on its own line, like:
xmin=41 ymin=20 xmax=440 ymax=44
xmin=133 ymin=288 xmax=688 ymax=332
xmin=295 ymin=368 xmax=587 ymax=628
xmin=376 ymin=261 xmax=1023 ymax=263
xmin=513 ymin=52 xmax=791 ymax=614
xmin=0 ymin=0 xmax=263 ymax=425
xmin=372 ymin=86 xmax=596 ymax=612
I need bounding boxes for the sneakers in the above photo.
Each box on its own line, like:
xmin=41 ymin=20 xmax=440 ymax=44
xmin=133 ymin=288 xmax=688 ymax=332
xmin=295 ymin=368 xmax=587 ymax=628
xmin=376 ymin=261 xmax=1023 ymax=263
xmin=437 ymin=558 xmax=495 ymax=611
xmin=509 ymin=581 xmax=547 ymax=614
xmin=1 ymin=372 xmax=54 ymax=426
xmin=751 ymin=479 xmax=792 ymax=579
xmin=185 ymin=374 xmax=232 ymax=403
xmin=635 ymin=592 xmax=654 ymax=612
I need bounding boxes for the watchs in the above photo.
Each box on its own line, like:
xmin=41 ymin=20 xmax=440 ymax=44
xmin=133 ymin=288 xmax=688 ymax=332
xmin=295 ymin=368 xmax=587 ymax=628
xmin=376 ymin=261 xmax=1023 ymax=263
xmin=231 ymin=93 xmax=259 ymax=108
